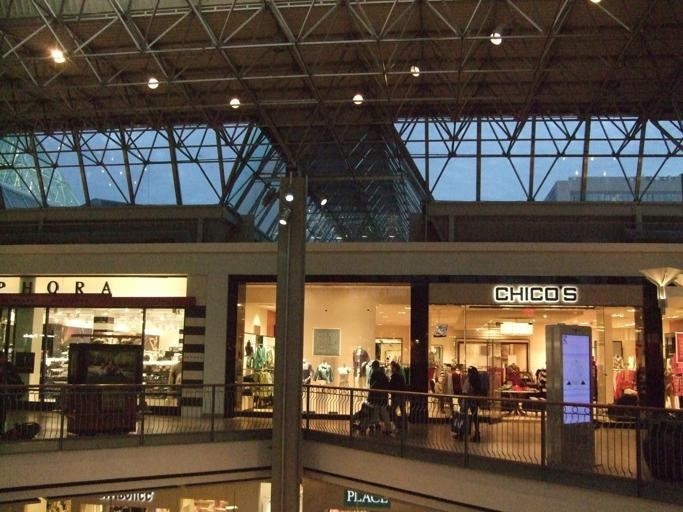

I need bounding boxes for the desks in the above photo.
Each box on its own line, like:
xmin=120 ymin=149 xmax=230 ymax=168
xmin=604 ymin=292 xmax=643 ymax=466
xmin=495 ymin=389 xmax=540 ymax=416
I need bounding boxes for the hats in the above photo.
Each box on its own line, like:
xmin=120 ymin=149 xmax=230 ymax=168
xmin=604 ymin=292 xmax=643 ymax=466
xmin=369 ymin=360 xmax=380 ymax=367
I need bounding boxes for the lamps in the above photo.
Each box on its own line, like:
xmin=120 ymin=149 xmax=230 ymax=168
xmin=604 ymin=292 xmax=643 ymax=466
xmin=500 ymin=321 xmax=533 ymax=337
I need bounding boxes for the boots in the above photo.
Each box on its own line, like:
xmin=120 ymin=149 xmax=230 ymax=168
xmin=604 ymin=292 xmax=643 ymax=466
xmin=453 ymin=434 xmax=464 ymax=440
xmin=470 ymin=433 xmax=481 ymax=442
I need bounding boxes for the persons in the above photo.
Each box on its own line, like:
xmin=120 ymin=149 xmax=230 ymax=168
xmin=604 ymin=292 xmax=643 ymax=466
xmin=337 ymin=362 xmax=352 ymax=389
xmin=442 ymin=362 xmax=455 ymax=413
xmin=452 ymin=363 xmax=465 ymax=411
xmin=0 ymin=349 xmax=26 ymax=433
xmin=301 ymin=358 xmax=315 ymax=387
xmin=311 ymin=360 xmax=336 ymax=385
xmin=351 ymin=345 xmax=370 ymax=388
xmin=366 ymin=360 xmax=395 ymax=437
xmin=505 ymin=362 xmax=520 ymax=380
xmin=449 ymin=365 xmax=483 ymax=442
xmin=434 ymin=377 xmax=447 ymax=412
xmin=384 ymin=360 xmax=409 ymax=431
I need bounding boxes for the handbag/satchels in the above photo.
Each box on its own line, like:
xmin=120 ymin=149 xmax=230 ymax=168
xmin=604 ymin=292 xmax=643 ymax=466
xmin=451 ymin=412 xmax=465 ymax=434
xmin=7 ymin=375 xmax=26 ymax=397
xmin=362 ymin=401 xmax=379 ymax=423
